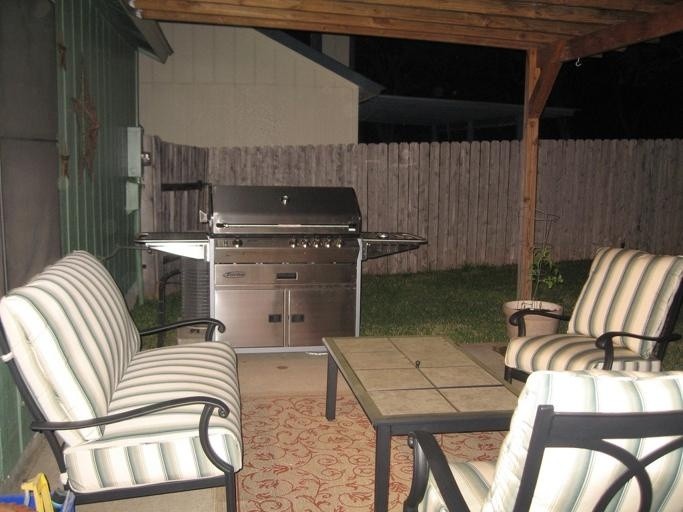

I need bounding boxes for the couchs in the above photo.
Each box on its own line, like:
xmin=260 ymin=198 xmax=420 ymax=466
xmin=0 ymin=249 xmax=241 ymax=512
xmin=505 ymin=248 xmax=683 ymax=385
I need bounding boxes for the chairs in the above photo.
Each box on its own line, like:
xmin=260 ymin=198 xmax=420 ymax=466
xmin=425 ymin=372 xmax=683 ymax=512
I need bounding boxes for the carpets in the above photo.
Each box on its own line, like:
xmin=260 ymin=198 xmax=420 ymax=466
xmin=240 ymin=393 xmax=505 ymax=511
